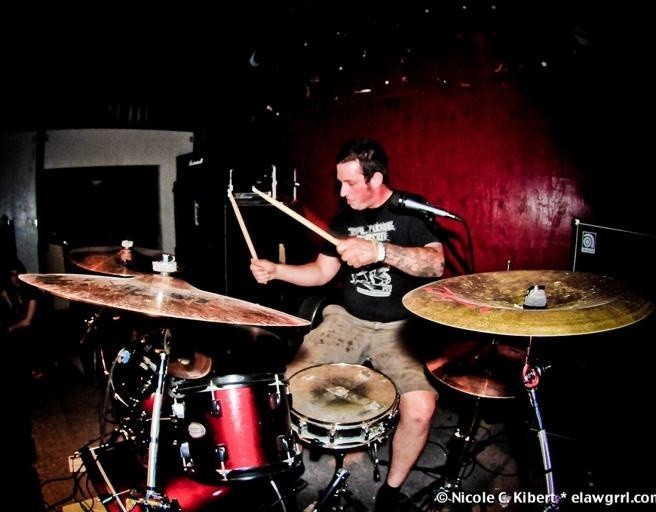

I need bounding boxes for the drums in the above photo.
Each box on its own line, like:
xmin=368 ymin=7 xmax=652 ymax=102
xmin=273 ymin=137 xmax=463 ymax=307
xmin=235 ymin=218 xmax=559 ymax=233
xmin=290 ymin=363 xmax=399 ymax=449
xmin=212 ymin=372 xmax=295 ymax=479
xmin=107 ymin=333 xmax=213 ymax=419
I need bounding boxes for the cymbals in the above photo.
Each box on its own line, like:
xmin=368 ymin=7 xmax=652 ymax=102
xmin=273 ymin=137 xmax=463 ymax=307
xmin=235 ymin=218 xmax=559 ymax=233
xmin=71 ymin=245 xmax=176 ymax=277
xmin=19 ymin=272 xmax=312 ymax=330
xmin=400 ymin=271 xmax=652 ymax=337
xmin=427 ymin=343 xmax=526 ymax=399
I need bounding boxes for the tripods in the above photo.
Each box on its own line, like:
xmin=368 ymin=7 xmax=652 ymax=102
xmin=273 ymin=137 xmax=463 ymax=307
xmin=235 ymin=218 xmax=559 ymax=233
xmin=411 ymin=401 xmax=489 ymax=512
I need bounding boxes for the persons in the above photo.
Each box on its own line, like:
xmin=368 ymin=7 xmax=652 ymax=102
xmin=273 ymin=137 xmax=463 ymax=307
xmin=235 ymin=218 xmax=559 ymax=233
xmin=248 ymin=137 xmax=446 ymax=511
xmin=0 ymin=258 xmax=54 ymax=380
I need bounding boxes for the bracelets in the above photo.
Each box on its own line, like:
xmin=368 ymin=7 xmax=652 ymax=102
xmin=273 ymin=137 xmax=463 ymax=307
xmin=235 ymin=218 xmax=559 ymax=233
xmin=371 ymin=238 xmax=386 ymax=265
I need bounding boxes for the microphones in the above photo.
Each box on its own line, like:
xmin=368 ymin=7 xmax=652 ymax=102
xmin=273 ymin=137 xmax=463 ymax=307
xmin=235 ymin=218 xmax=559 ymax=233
xmin=391 ymin=192 xmax=462 ymax=221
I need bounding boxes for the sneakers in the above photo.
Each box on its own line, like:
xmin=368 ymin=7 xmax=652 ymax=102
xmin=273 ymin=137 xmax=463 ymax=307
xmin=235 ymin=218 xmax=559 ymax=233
xmin=376 ymin=492 xmax=422 ymax=512
